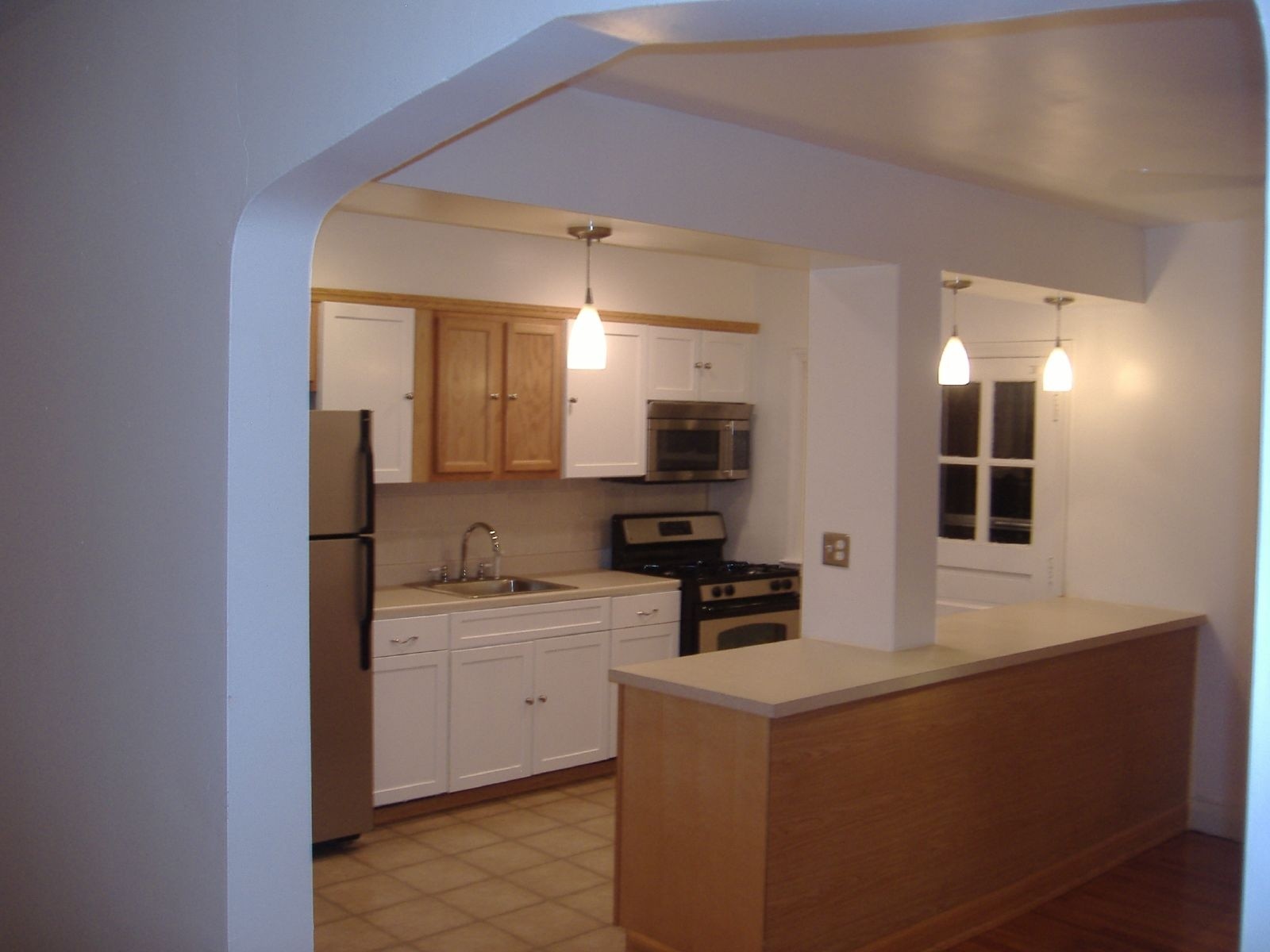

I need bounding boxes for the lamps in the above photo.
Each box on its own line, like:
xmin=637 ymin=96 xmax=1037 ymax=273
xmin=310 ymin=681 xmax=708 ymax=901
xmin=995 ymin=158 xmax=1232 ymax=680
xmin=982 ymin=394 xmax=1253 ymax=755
xmin=1043 ymin=297 xmax=1076 ymax=392
xmin=938 ymin=278 xmax=971 ymax=386
xmin=566 ymin=225 xmax=611 ymax=370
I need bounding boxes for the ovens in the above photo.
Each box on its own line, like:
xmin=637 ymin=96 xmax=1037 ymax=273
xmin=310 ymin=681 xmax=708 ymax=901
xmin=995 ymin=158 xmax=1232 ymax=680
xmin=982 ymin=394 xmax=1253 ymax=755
xmin=680 ymin=597 xmax=803 ymax=657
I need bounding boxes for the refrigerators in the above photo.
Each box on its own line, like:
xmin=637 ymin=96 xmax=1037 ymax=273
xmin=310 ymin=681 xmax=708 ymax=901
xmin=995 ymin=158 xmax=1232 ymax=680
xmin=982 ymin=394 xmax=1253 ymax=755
xmin=309 ymin=408 xmax=375 ymax=846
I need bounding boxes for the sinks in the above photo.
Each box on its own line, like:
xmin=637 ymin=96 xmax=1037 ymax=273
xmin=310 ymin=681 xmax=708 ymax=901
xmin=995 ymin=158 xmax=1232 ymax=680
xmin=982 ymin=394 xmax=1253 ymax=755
xmin=402 ymin=574 xmax=578 ymax=599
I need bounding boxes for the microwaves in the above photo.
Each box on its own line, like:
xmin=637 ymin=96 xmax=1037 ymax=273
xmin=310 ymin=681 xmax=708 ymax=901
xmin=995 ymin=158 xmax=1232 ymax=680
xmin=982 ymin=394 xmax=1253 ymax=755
xmin=645 ymin=401 xmax=752 ymax=482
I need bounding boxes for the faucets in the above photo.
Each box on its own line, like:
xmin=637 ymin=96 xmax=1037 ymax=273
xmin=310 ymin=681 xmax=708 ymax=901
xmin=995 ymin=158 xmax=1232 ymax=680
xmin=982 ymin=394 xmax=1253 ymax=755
xmin=460 ymin=521 xmax=501 ymax=582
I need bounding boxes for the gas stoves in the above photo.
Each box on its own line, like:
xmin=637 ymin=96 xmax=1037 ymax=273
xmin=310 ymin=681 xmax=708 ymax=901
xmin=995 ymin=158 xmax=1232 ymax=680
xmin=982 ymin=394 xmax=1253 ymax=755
xmin=610 ymin=512 xmax=802 ymax=603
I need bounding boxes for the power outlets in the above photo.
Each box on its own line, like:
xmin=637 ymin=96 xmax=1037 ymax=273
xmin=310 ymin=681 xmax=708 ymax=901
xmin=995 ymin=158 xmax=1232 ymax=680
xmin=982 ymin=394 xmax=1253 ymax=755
xmin=823 ymin=532 xmax=850 ymax=567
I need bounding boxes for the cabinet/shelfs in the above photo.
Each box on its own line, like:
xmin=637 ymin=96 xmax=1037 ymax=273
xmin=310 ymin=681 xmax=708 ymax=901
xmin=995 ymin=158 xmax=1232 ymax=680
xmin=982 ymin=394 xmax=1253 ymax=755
xmin=449 ymin=597 xmax=611 ymax=792
xmin=610 ymin=589 xmax=682 ymax=760
xmin=560 ymin=318 xmax=646 ymax=477
xmin=319 ymin=301 xmax=415 ymax=485
xmin=372 ymin=615 xmax=449 ymax=806
xmin=434 ymin=311 xmax=561 ymax=472
xmin=645 ymin=325 xmax=756 ymax=403
